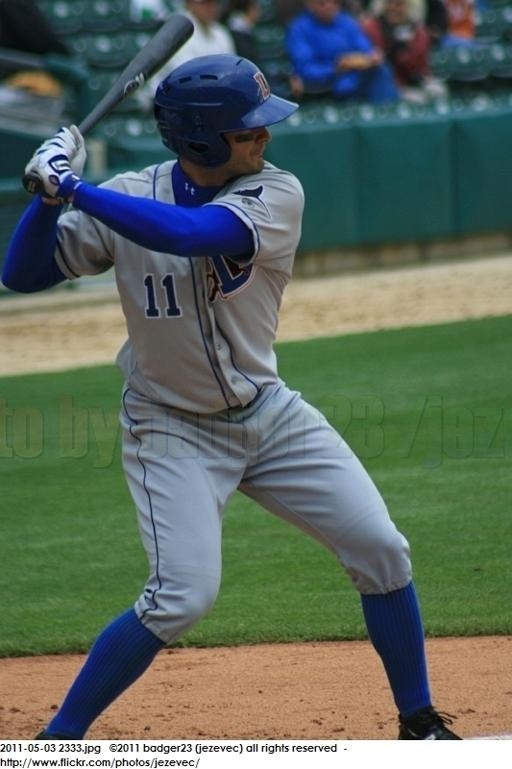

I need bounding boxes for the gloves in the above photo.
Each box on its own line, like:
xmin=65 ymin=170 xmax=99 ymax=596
xmin=24 ymin=125 xmax=87 ymax=199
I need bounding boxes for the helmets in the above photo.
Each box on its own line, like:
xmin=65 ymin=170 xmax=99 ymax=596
xmin=155 ymin=55 xmax=299 ymax=166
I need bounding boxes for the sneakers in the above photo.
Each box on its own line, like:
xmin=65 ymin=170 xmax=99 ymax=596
xmin=397 ymin=707 xmax=464 ymax=740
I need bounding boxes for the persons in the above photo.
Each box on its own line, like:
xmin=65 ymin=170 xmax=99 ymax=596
xmin=0 ymin=55 xmax=464 ymax=740
xmin=0 ymin=0 xmax=512 ymax=130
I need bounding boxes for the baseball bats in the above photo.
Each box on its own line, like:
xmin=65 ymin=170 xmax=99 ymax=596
xmin=23 ymin=15 xmax=194 ymax=193
